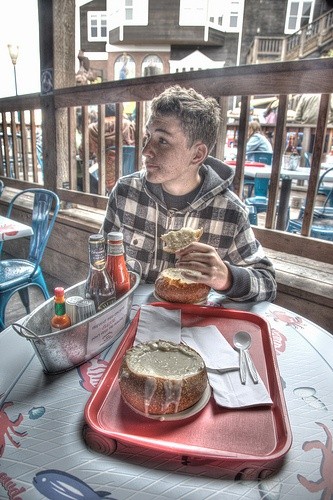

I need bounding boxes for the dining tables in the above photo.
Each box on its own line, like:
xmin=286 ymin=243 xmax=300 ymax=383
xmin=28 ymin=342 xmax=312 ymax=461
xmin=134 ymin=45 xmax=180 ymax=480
xmin=0 ymin=284 xmax=333 ymax=500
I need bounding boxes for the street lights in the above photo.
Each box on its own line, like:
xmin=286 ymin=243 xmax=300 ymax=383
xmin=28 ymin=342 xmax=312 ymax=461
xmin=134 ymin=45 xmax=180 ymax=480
xmin=5 ymin=41 xmax=19 ymax=97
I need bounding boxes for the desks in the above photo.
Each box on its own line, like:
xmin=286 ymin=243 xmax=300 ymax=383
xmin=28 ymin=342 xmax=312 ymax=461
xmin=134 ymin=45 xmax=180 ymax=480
xmin=225 ymin=161 xmax=333 ymax=199
xmin=0 ymin=213 xmax=33 ymax=241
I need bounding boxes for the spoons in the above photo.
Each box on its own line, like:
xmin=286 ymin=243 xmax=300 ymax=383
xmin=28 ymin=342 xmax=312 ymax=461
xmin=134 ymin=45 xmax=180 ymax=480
xmin=235 ymin=330 xmax=260 ymax=384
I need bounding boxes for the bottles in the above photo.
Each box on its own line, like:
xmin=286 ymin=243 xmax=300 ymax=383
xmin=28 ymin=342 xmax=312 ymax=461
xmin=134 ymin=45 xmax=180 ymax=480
xmin=283 ymin=135 xmax=300 ymax=155
xmin=82 ymin=234 xmax=116 ymax=315
xmin=50 ymin=286 xmax=71 ymax=335
xmin=106 ymin=231 xmax=131 ymax=300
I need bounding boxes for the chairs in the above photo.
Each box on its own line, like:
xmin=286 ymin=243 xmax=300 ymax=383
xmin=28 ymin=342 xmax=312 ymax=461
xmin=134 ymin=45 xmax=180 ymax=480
xmin=107 ymin=145 xmax=136 ymax=177
xmin=0 ymin=134 xmax=22 ymax=178
xmin=0 ymin=188 xmax=59 ymax=331
xmin=229 ymin=147 xmax=333 ymax=241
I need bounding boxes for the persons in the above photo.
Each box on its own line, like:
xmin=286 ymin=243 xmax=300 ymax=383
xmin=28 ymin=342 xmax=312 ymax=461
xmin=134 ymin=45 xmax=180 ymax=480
xmin=99 ymin=84 xmax=277 ymax=302
xmin=295 ymin=91 xmax=331 ymax=155
xmin=245 ymin=119 xmax=274 ymax=162
xmin=78 ymin=102 xmax=136 ymax=196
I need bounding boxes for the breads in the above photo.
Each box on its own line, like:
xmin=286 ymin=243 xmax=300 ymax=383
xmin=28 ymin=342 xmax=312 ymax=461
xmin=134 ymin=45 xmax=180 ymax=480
xmin=154 ymin=268 xmax=211 ymax=305
xmin=118 ymin=339 xmax=207 ymax=415
xmin=160 ymin=227 xmax=204 ymax=254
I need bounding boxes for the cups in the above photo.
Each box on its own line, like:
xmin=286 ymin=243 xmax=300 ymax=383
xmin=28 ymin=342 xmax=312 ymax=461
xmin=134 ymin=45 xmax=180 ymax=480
xmin=65 ymin=295 xmax=83 ymax=327
xmin=75 ymin=299 xmax=96 ymax=326
xmin=283 ymin=155 xmax=301 ymax=172
